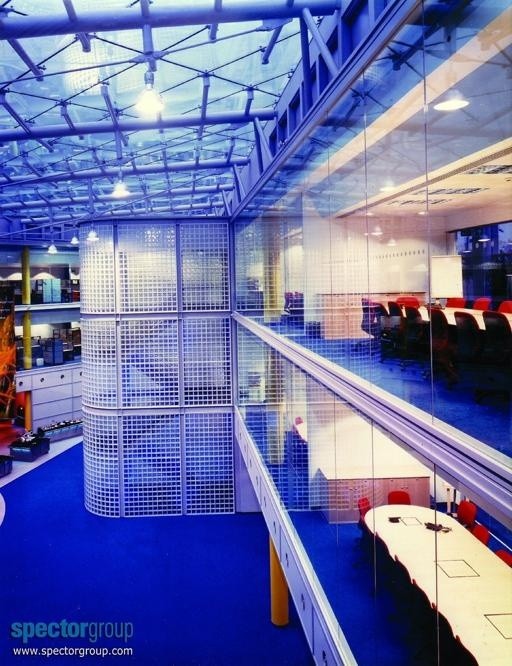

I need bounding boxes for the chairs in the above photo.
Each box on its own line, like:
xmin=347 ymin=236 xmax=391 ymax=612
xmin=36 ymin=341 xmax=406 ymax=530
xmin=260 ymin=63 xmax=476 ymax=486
xmin=361 ymin=297 xmax=511 ymax=404
xmin=283 ymin=291 xmax=304 ymax=330
xmin=357 ymin=490 xmax=512 ymax=666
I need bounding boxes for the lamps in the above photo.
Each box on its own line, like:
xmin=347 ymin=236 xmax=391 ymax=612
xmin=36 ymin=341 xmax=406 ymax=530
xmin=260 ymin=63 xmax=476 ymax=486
xmin=46 ymin=216 xmax=99 ymax=254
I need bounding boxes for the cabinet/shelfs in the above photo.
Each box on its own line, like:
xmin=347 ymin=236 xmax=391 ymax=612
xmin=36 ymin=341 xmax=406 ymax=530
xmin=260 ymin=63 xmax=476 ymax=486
xmin=315 ymin=290 xmax=428 ymax=340
xmin=317 ymin=466 xmax=431 ymax=526
